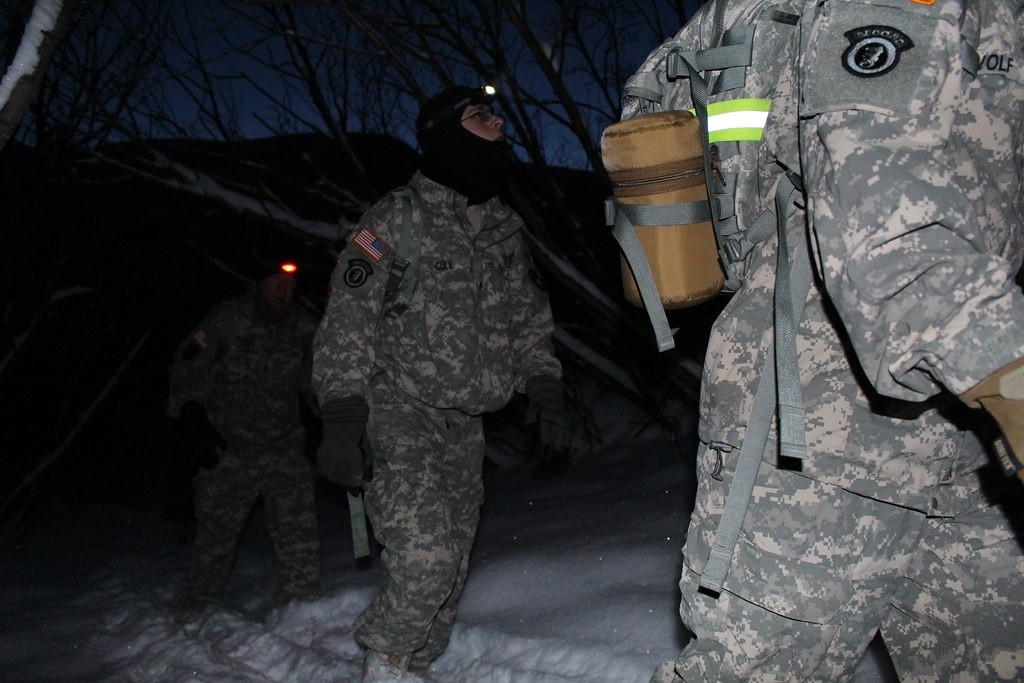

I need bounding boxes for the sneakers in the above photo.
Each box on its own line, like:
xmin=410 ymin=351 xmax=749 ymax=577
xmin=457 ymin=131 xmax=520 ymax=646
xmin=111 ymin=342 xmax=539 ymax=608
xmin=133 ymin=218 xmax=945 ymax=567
xmin=360 ymin=645 xmax=432 ymax=683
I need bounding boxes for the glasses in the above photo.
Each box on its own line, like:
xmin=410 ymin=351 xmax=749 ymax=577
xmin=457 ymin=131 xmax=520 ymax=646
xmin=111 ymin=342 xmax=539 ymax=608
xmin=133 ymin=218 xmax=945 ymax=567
xmin=463 ymin=109 xmax=499 ymax=122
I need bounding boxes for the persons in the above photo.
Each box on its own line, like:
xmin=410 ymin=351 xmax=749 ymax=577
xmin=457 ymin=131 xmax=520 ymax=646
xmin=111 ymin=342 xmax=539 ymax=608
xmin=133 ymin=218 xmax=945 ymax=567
xmin=312 ymin=83 xmax=564 ymax=683
xmin=169 ymin=261 xmax=320 ymax=627
xmin=621 ymin=0 xmax=1024 ymax=683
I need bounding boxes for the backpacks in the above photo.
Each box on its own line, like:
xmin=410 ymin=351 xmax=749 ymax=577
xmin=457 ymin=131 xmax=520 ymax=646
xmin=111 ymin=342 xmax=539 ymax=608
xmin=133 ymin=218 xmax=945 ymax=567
xmin=602 ymin=1 xmax=982 ymax=311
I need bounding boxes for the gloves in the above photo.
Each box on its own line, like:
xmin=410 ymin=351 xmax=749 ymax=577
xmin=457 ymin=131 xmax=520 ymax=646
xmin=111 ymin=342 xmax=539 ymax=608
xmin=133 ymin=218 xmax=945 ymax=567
xmin=316 ymin=396 xmax=372 ymax=489
xmin=181 ymin=404 xmax=226 ymax=470
xmin=524 ymin=374 xmax=573 ymax=454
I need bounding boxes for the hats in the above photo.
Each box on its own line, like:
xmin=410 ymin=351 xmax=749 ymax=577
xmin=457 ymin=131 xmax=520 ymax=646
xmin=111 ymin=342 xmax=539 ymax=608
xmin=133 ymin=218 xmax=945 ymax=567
xmin=244 ymin=256 xmax=291 ymax=292
